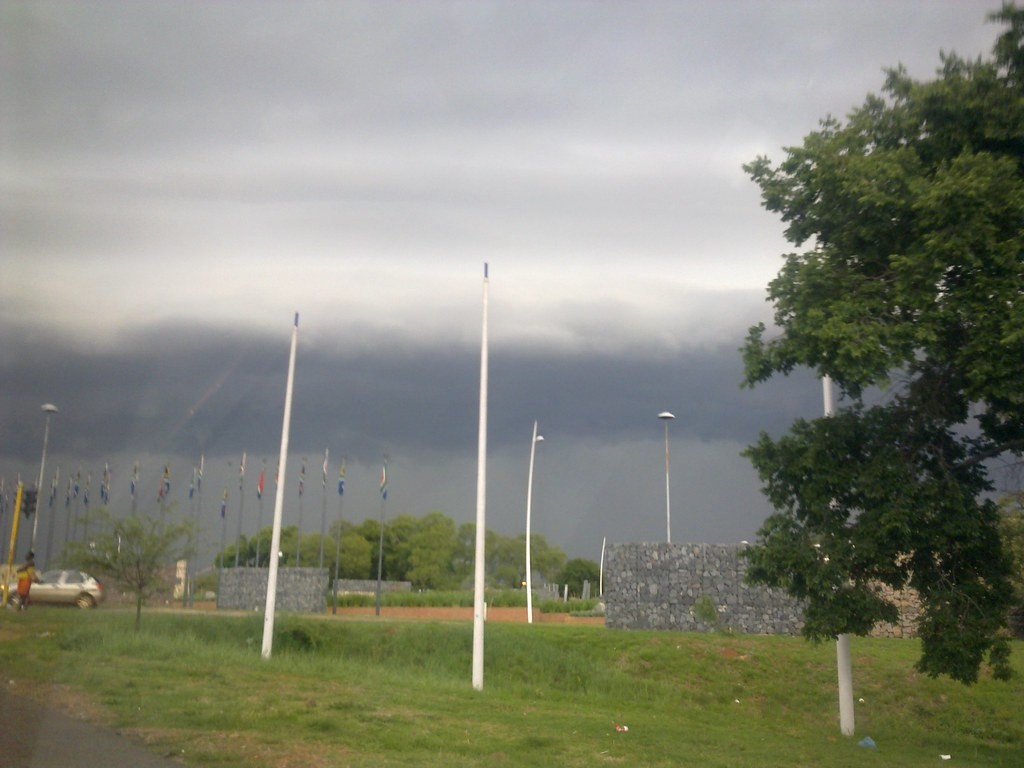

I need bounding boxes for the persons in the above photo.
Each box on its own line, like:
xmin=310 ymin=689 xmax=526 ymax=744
xmin=17 ymin=551 xmax=37 ymax=611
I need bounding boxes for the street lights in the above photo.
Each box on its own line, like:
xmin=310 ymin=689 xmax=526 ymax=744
xmin=29 ymin=402 xmax=58 ymax=555
xmin=656 ymin=410 xmax=676 ymax=543
xmin=523 ymin=421 xmax=547 ymax=624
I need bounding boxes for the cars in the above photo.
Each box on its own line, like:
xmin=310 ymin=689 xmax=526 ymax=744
xmin=0 ymin=568 xmax=108 ymax=608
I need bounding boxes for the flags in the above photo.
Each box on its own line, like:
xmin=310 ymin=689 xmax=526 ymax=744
xmin=338 ymin=462 xmax=345 ymax=495
xmin=380 ymin=461 xmax=386 ymax=502
xmin=49 ymin=450 xmax=328 ymax=519
